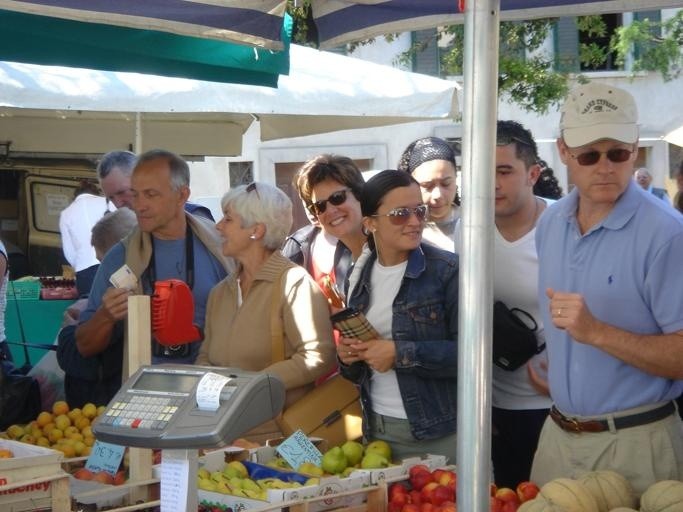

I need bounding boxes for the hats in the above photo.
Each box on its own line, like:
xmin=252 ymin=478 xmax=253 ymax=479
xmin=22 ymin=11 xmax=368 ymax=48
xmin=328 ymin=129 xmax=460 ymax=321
xmin=559 ymin=83 xmax=638 ymax=148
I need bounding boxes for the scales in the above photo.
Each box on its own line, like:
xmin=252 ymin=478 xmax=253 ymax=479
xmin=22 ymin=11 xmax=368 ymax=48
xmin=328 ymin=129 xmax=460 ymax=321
xmin=91 ymin=363 xmax=286 ymax=512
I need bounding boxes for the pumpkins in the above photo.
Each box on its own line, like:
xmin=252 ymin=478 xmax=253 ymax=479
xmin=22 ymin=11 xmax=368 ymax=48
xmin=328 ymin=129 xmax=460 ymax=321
xmin=517 ymin=471 xmax=683 ymax=512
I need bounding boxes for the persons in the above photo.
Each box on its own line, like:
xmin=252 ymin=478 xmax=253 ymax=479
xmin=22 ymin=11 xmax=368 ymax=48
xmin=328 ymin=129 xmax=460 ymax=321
xmin=1 ymin=83 xmax=683 ymax=490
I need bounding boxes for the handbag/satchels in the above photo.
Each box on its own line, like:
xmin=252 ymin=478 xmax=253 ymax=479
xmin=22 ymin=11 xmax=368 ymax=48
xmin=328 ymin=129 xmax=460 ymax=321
xmin=493 ymin=301 xmax=538 ymax=372
xmin=274 ymin=373 xmax=363 ymax=455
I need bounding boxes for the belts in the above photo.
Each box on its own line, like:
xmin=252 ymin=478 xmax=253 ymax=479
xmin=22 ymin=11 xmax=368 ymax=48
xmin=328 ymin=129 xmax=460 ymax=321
xmin=549 ymin=400 xmax=676 ymax=433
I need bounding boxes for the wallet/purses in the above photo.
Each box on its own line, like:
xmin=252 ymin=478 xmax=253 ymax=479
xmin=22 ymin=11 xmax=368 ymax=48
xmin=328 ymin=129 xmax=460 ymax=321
xmin=329 ymin=307 xmax=382 ymax=343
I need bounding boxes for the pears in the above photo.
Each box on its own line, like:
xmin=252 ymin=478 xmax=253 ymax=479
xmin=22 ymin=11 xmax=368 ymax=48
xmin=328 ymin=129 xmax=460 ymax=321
xmin=198 ymin=440 xmax=397 ymax=501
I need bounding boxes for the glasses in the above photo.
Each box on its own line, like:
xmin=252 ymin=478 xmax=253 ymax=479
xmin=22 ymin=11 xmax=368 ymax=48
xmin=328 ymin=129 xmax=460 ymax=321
xmin=496 ymin=135 xmax=531 ymax=147
xmin=565 ymin=145 xmax=635 ymax=165
xmin=308 ymin=188 xmax=351 ymax=215
xmin=246 ymin=183 xmax=260 ymax=200
xmin=370 ymin=204 xmax=429 ymax=225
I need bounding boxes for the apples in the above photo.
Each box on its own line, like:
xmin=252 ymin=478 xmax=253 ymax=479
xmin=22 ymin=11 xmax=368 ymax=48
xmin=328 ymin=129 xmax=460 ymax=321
xmin=93 ymin=471 xmax=115 ymax=485
xmin=1 ymin=401 xmax=104 ymax=459
xmin=115 ymin=471 xmax=128 ymax=485
xmin=75 ymin=469 xmax=93 ymax=481
xmin=389 ymin=465 xmax=540 ymax=512
